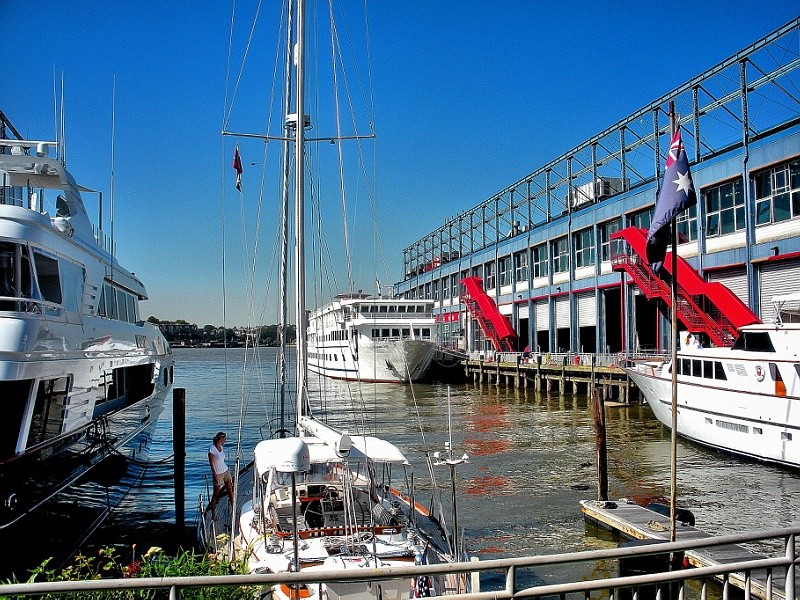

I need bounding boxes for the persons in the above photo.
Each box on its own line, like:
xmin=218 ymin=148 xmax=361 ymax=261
xmin=207 ymin=431 xmax=237 ymax=522
xmin=523 ymin=344 xmax=532 ymax=364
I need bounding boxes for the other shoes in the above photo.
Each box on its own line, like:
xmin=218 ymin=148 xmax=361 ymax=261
xmin=211 ymin=511 xmax=217 ymax=520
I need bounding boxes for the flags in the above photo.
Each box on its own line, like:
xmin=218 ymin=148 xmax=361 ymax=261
xmin=644 ymin=123 xmax=700 ymax=265
xmin=234 ymin=144 xmax=243 ymax=194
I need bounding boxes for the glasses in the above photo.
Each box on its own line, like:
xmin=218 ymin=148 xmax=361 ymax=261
xmin=222 ymin=438 xmax=227 ymax=440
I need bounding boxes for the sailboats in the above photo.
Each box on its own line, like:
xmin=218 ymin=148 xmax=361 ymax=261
xmin=194 ymin=0 xmax=475 ymax=600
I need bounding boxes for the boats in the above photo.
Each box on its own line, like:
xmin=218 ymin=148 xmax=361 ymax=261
xmin=300 ymin=279 xmax=437 ymax=383
xmin=1 ymin=65 xmax=177 ymax=535
xmin=618 ymin=290 xmax=800 ymax=472
